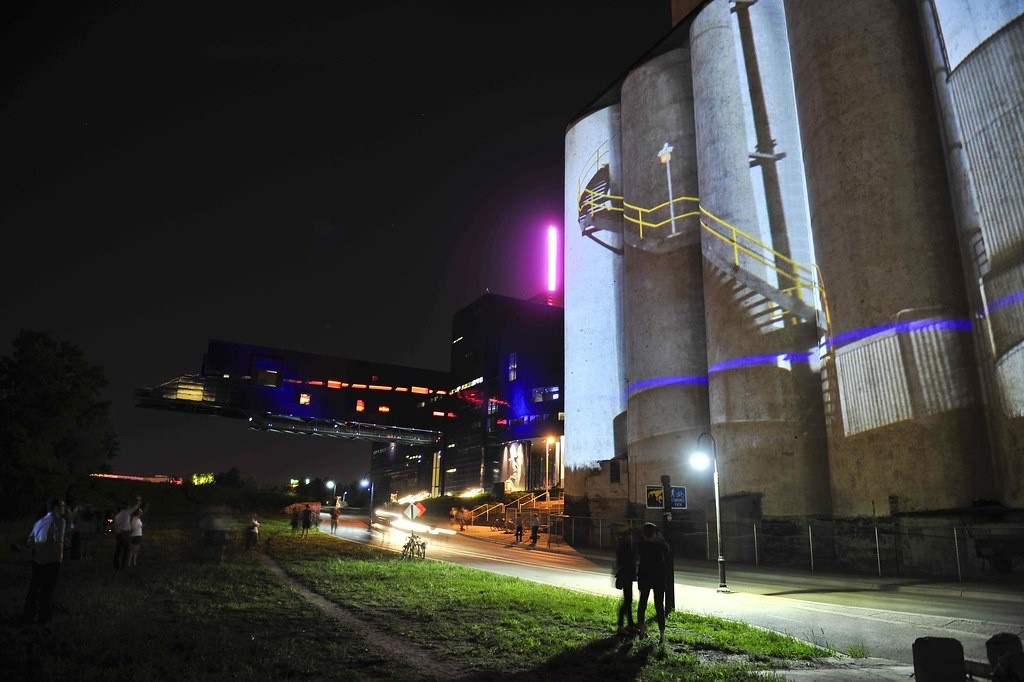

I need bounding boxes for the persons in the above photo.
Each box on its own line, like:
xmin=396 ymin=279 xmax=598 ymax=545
xmin=516 ymin=513 xmax=539 ymax=544
xmin=198 ymin=505 xmax=231 ymax=561
xmin=505 ymin=443 xmax=524 ymax=492
xmin=113 ymin=495 xmax=143 ymax=576
xmin=615 ymin=523 xmax=668 ymax=642
xmin=330 ymin=506 xmax=340 ymax=529
xmin=245 ymin=513 xmax=261 ymax=548
xmin=21 ymin=497 xmax=97 ymax=625
xmin=449 ymin=507 xmax=471 ymax=526
xmin=291 ymin=504 xmax=313 ymax=539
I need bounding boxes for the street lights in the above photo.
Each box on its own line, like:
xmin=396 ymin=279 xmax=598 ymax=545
xmin=327 ymin=481 xmax=336 ymax=496
xmin=545 ymin=436 xmax=556 ymax=503
xmin=361 ymin=471 xmax=374 ymax=532
xmin=688 ymin=433 xmax=731 ymax=594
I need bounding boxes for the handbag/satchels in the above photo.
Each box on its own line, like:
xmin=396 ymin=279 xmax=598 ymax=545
xmin=616 ymin=577 xmax=622 ymax=589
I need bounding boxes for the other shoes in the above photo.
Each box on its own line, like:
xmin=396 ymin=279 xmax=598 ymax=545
xmin=619 ymin=626 xmax=640 ymax=635
xmin=661 ymin=629 xmax=664 ymax=634
xmin=638 ymin=624 xmax=646 ymax=634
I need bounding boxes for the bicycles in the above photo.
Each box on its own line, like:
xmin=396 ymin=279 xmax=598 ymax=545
xmin=402 ymin=535 xmax=427 ymax=560
